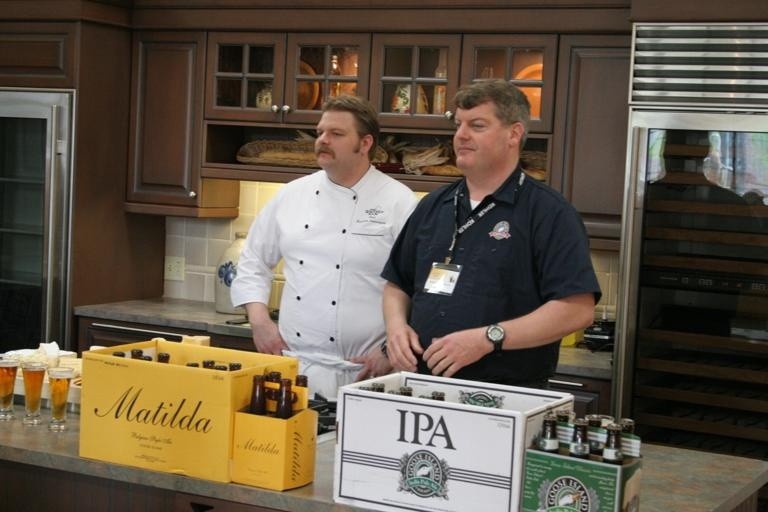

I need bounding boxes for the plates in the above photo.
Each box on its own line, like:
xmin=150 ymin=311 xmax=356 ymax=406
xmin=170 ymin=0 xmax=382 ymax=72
xmin=512 ymin=62 xmax=543 ymax=119
xmin=297 ymin=59 xmax=319 ymax=111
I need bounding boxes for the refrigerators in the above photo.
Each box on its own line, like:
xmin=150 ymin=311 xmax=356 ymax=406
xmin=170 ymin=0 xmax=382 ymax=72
xmin=1 ymin=88 xmax=74 ymax=359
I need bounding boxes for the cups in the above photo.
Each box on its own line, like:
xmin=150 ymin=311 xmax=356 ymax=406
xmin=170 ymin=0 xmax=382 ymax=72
xmin=0 ymin=360 xmax=75 ymax=432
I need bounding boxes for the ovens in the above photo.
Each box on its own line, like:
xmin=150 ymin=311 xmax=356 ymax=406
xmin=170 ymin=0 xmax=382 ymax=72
xmin=608 ymin=20 xmax=768 ymax=464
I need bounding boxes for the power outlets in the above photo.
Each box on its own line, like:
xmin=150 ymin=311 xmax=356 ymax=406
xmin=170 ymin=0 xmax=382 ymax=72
xmin=164 ymin=255 xmax=185 ymax=282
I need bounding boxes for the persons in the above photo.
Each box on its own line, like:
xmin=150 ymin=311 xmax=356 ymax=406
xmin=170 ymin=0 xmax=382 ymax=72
xmin=226 ymin=93 xmax=426 ymax=407
xmin=378 ymin=76 xmax=604 ymax=401
xmin=640 ymin=129 xmax=764 ymax=352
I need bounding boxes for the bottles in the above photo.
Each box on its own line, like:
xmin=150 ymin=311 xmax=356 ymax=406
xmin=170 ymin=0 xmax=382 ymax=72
xmin=322 ymin=55 xmax=341 ymax=111
xmin=247 ymin=370 xmax=309 ymax=420
xmin=433 ymin=46 xmax=447 ymax=115
xmin=539 ymin=408 xmax=635 ymax=463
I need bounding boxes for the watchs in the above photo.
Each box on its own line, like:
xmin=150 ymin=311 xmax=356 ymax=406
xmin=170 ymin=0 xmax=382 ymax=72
xmin=485 ymin=320 xmax=506 ymax=352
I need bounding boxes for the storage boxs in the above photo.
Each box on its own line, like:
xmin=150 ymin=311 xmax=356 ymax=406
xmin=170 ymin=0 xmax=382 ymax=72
xmin=78 ymin=340 xmax=296 ymax=484
xmin=518 ymin=442 xmax=645 ymax=512
xmin=335 ymin=370 xmax=574 ymax=512
xmin=235 ymin=402 xmax=318 ymax=493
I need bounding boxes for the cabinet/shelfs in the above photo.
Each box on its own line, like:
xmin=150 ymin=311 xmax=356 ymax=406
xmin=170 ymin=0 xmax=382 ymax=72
xmin=368 ymin=31 xmax=560 ymax=193
xmin=127 ymin=30 xmax=240 ymax=219
xmin=545 ymin=32 xmax=642 ymax=252
xmin=206 ymin=332 xmax=255 ymax=352
xmin=0 ymin=21 xmax=132 ymax=88
xmin=200 ymin=30 xmax=372 ymax=183
xmin=547 ymin=373 xmax=610 ymax=417
xmin=73 ymin=315 xmax=207 ymax=359
